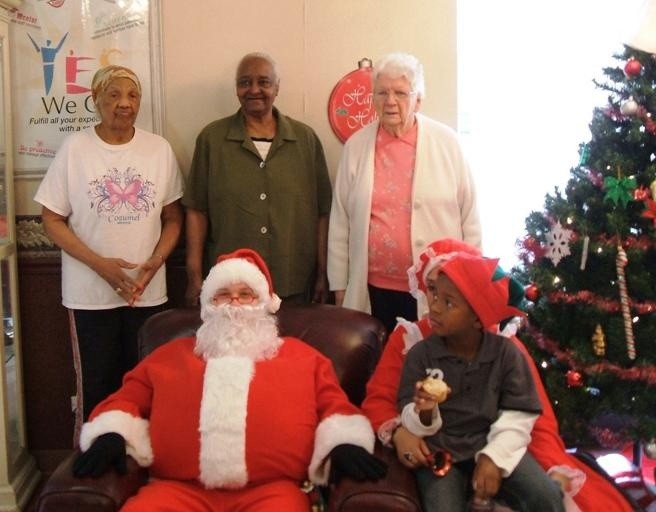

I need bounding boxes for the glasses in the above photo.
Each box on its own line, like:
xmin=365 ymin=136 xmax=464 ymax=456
xmin=212 ymin=291 xmax=259 ymax=304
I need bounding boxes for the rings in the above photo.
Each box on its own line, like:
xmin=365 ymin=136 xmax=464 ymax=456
xmin=115 ymin=287 xmax=122 ymax=293
xmin=404 ymin=451 xmax=412 ymax=460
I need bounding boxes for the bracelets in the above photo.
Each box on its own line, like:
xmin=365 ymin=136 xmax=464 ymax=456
xmin=151 ymin=254 xmax=164 ymax=263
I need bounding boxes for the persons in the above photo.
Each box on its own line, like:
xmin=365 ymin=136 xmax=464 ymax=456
xmin=34 ymin=68 xmax=187 ymax=447
xmin=362 ymin=240 xmax=634 ymax=511
xmin=181 ymin=50 xmax=333 ymax=307
xmin=400 ymin=253 xmax=565 ymax=512
xmin=326 ymin=52 xmax=481 ymax=338
xmin=69 ymin=246 xmax=389 ymax=512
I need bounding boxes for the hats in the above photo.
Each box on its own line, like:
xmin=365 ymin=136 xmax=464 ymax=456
xmin=408 ymin=240 xmax=526 ymax=331
xmin=203 ymin=248 xmax=282 ymax=314
xmin=92 ymin=65 xmax=141 ymax=98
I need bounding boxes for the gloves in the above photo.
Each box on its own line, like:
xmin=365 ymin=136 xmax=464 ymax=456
xmin=72 ymin=433 xmax=129 ymax=479
xmin=327 ymin=444 xmax=389 ymax=485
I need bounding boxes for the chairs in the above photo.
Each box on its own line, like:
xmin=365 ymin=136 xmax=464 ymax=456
xmin=32 ymin=303 xmax=419 ymax=512
